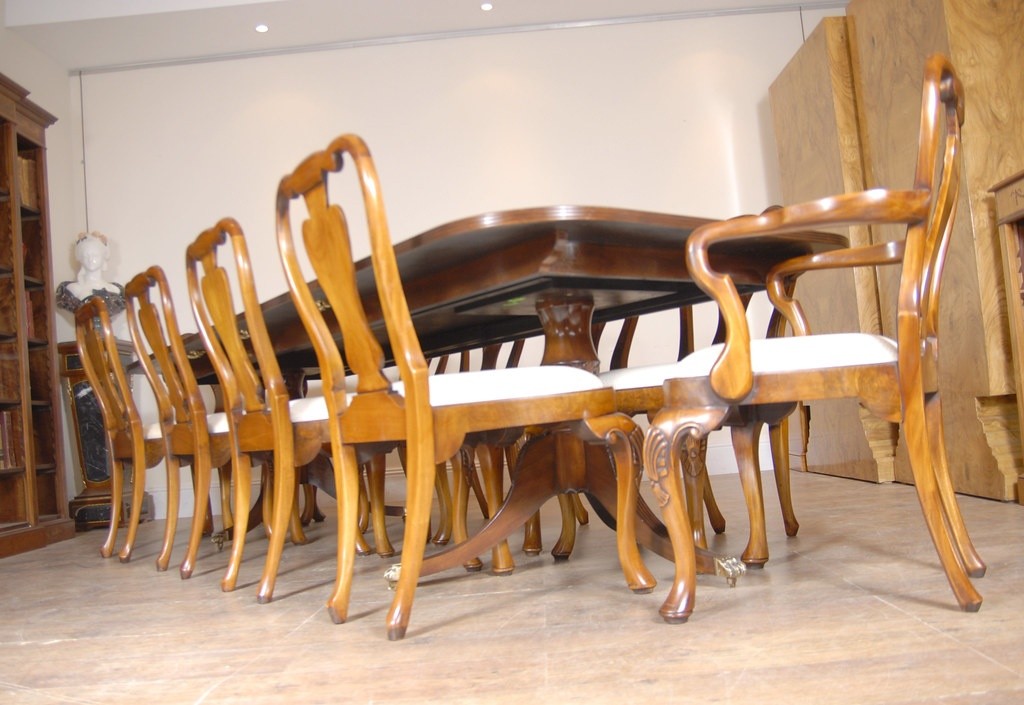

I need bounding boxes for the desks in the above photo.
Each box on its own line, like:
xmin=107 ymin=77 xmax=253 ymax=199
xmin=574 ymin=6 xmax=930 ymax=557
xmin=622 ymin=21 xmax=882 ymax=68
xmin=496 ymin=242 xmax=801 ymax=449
xmin=124 ymin=206 xmax=813 ymax=589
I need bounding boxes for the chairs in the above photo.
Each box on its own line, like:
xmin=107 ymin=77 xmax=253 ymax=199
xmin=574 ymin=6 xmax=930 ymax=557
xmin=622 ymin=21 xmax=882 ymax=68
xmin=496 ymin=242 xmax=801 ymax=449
xmin=302 ymin=258 xmax=800 ymax=540
xmin=185 ymin=219 xmax=451 ymax=599
xmin=125 ymin=268 xmax=370 ymax=592
xmin=274 ymin=135 xmax=657 ymax=639
xmin=72 ymin=295 xmax=255 ymax=564
xmin=641 ymin=52 xmax=986 ymax=623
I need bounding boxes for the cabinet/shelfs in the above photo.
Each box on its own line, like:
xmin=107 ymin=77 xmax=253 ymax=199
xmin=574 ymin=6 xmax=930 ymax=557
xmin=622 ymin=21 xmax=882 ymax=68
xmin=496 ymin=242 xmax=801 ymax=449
xmin=0 ymin=72 xmax=77 ymax=560
xmin=770 ymin=0 xmax=1024 ymax=502
